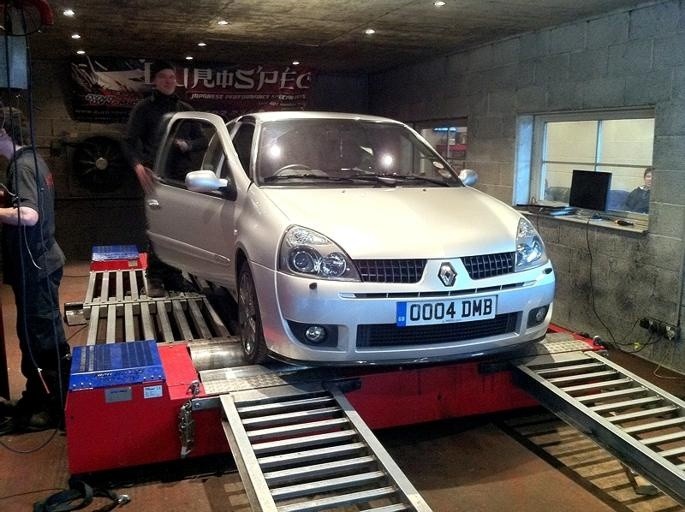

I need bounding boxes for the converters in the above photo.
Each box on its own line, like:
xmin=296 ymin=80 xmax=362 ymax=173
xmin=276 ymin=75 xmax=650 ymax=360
xmin=639 ymin=319 xmax=649 ymax=329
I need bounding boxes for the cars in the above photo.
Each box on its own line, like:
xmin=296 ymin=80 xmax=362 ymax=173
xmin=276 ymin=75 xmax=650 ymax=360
xmin=71 ymin=56 xmax=145 ymax=92
xmin=143 ymin=111 xmax=555 ymax=367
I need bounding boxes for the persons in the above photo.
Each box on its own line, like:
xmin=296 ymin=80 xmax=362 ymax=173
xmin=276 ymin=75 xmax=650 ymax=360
xmin=0 ymin=108 xmax=72 ymax=433
xmin=618 ymin=167 xmax=652 ymax=215
xmin=123 ymin=63 xmax=209 ymax=297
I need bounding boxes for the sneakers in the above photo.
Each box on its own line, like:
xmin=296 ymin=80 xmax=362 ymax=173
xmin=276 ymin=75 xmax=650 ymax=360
xmin=146 ymin=276 xmax=165 ymax=298
xmin=1 ymin=416 xmax=17 ymax=436
xmin=27 ymin=408 xmax=50 ymax=431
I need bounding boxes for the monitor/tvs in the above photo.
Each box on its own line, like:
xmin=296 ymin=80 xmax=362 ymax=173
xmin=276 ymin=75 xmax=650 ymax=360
xmin=569 ymin=169 xmax=613 ymax=213
